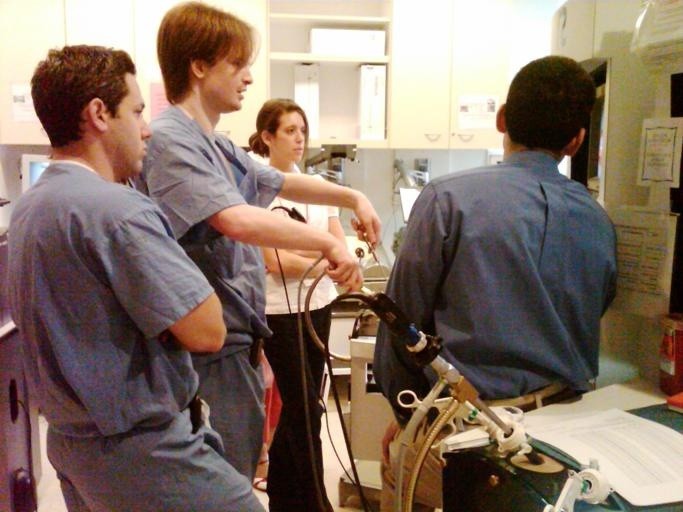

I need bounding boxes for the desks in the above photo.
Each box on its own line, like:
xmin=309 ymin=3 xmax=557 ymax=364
xmin=443 ymin=367 xmax=683 ymax=512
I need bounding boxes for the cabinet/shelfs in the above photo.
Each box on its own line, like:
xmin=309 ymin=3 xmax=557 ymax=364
xmin=268 ymin=1 xmax=390 ymax=149
xmin=0 ymin=0 xmax=135 ymax=146
xmin=135 ymin=0 xmax=268 ymax=149
xmin=390 ymin=1 xmax=511 ymax=151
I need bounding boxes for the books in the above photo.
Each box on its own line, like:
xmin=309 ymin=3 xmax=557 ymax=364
xmin=525 ymin=408 xmax=683 ymax=511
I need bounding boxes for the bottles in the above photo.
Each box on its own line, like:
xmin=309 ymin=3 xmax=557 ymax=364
xmin=658 ymin=315 xmax=683 ymax=395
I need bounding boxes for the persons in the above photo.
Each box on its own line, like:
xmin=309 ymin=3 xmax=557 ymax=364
xmin=135 ymin=0 xmax=380 ymax=483
xmin=247 ymin=97 xmax=348 ymax=512
xmin=373 ymin=56 xmax=618 ymax=427
xmin=6 ymin=44 xmax=264 ymax=512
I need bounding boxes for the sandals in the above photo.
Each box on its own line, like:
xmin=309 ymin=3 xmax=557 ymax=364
xmin=253 ymin=461 xmax=267 ymax=491
